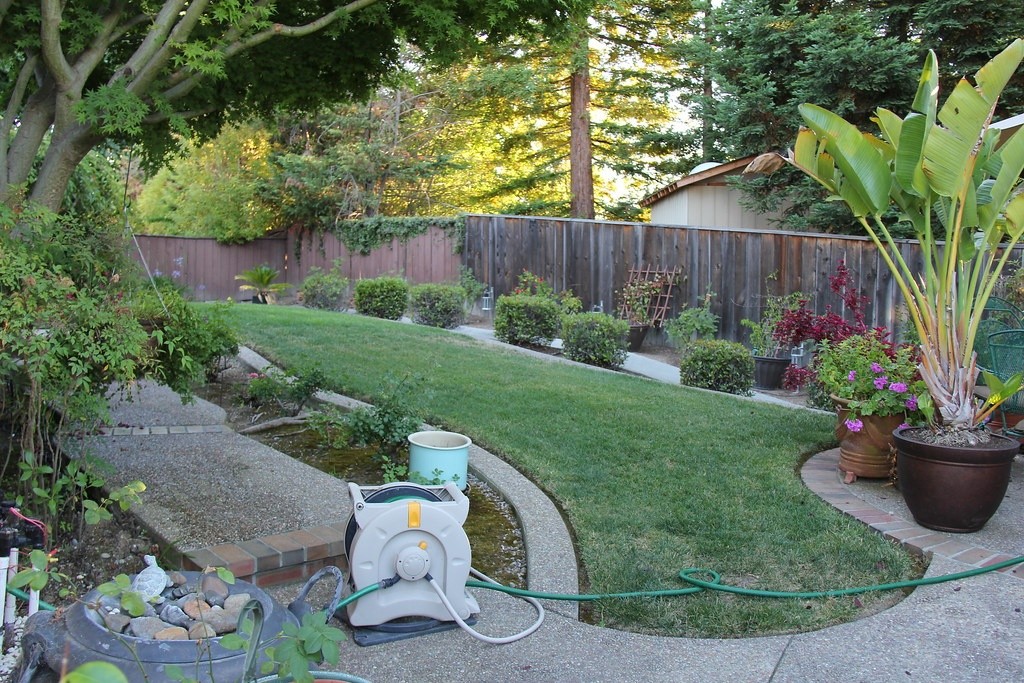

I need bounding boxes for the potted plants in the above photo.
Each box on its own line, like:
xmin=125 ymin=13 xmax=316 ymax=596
xmin=232 ymin=261 xmax=295 ymax=304
xmin=740 ymin=268 xmax=815 ymax=391
xmin=740 ymin=39 xmax=1024 ymax=535
xmin=622 ymin=277 xmax=663 ymax=352
xmin=103 ymin=287 xmax=176 ymax=378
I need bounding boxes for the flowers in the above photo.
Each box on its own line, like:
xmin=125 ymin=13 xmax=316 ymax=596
xmin=817 ymin=332 xmax=924 ymax=432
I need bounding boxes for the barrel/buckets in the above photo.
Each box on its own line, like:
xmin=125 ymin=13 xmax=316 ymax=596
xmin=407 ymin=431 xmax=472 ymax=492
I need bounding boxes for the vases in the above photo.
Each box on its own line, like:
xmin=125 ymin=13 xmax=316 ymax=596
xmin=407 ymin=431 xmax=472 ymax=492
xmin=829 ymin=391 xmax=904 ymax=483
